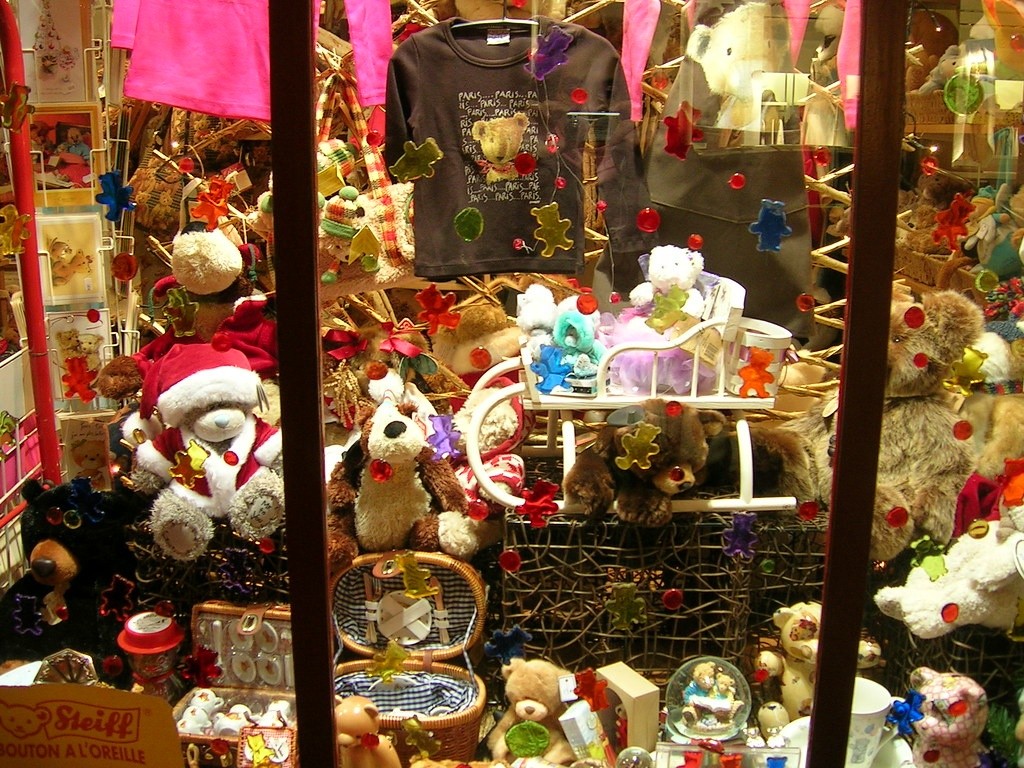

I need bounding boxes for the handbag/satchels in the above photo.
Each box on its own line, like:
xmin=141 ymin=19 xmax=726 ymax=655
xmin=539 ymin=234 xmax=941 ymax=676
xmin=247 ymin=74 xmax=414 ymax=301
xmin=122 ymin=145 xmax=185 ymax=235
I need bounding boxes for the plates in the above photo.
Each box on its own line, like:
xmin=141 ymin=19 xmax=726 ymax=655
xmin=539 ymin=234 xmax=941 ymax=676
xmin=768 ymin=714 xmax=915 ymax=768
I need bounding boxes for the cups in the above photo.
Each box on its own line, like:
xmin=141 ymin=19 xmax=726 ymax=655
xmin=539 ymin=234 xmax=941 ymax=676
xmin=845 ymin=676 xmax=905 ymax=768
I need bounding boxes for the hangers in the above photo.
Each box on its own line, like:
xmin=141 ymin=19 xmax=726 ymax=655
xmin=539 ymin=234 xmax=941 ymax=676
xmin=451 ymin=0 xmax=539 ymax=29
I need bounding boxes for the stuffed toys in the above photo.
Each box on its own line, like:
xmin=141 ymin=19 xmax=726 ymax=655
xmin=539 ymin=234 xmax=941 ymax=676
xmin=487 ymin=658 xmax=577 ymax=766
xmin=94 ymin=183 xmax=1024 ymax=644
xmin=685 ymin=2 xmax=845 ymax=146
